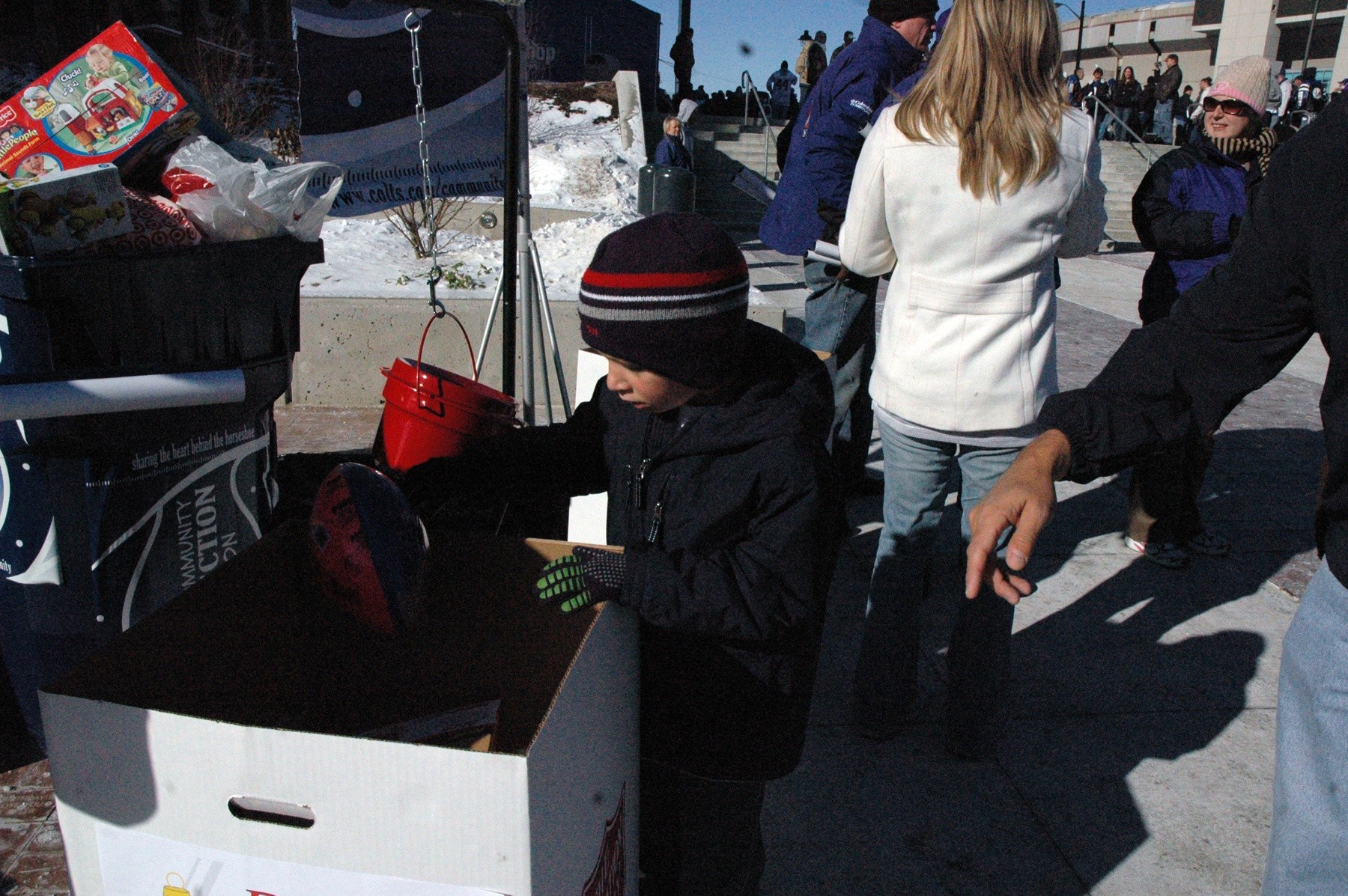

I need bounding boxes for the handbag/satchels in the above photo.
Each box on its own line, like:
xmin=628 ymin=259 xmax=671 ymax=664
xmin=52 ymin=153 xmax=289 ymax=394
xmin=163 ymin=133 xmax=344 ymax=244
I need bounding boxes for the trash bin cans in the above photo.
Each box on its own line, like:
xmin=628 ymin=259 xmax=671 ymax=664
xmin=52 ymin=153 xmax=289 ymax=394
xmin=1 ymin=234 xmax=326 ymax=639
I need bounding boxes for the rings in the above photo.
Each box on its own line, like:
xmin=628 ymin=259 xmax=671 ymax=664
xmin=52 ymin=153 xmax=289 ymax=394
xmin=845 ymin=278 xmax=850 ymax=283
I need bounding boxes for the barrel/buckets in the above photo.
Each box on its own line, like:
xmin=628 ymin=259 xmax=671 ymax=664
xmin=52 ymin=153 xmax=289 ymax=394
xmin=365 ymin=311 xmax=521 ymax=479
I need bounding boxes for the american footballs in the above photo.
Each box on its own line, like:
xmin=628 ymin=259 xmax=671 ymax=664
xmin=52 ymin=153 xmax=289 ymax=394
xmin=308 ymin=461 xmax=429 ymax=640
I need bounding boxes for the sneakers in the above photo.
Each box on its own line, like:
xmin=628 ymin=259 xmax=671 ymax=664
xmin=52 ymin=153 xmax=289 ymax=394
xmin=1125 ymin=531 xmax=1189 ymax=566
xmin=1188 ymin=525 xmax=1231 ymax=554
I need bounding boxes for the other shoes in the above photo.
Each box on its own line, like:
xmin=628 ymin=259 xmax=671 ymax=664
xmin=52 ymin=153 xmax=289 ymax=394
xmin=941 ymin=695 xmax=988 ymax=763
xmin=846 ymin=688 xmax=899 ymax=740
xmin=857 ymin=468 xmax=889 ymax=496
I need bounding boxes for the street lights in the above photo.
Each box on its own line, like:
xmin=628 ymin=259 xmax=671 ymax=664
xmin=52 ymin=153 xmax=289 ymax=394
xmin=1054 ymin=0 xmax=1085 ymax=69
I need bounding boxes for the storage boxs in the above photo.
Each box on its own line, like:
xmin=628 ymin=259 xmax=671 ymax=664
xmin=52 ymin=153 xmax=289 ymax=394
xmin=0 ymin=19 xmax=201 ymax=184
xmin=36 ymin=537 xmax=645 ymax=896
xmin=0 ymin=161 xmax=136 ymax=257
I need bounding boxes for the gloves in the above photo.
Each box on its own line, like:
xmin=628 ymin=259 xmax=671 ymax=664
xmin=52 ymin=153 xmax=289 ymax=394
xmin=407 ymin=456 xmax=483 ymax=513
xmin=536 ymin=545 xmax=631 ymax=613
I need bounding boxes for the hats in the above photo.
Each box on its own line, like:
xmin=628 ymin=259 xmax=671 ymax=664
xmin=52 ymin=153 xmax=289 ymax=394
xmin=1207 ymin=56 xmax=1272 ymax=116
xmin=1164 ymin=53 xmax=1179 ymax=62
xmin=845 ymin=30 xmax=856 ymax=39
xmin=577 ymin=212 xmax=750 ymax=388
xmin=868 ymin=0 xmax=940 ymax=24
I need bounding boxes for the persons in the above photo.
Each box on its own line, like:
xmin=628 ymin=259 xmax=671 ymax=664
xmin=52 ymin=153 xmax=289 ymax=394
xmin=654 ymin=28 xmax=1348 ymax=167
xmin=83 ymin=44 xmax=141 ymax=99
xmin=396 ymin=210 xmax=836 ymax=896
xmin=965 ymin=56 xmax=1348 ymax=896
xmin=757 ymin=0 xmax=1108 ymax=761
xmin=21 ymin=154 xmax=54 ymax=178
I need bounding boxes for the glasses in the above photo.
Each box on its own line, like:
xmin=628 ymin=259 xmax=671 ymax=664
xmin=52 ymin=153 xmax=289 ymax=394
xmin=1203 ymin=97 xmax=1250 ymax=116
